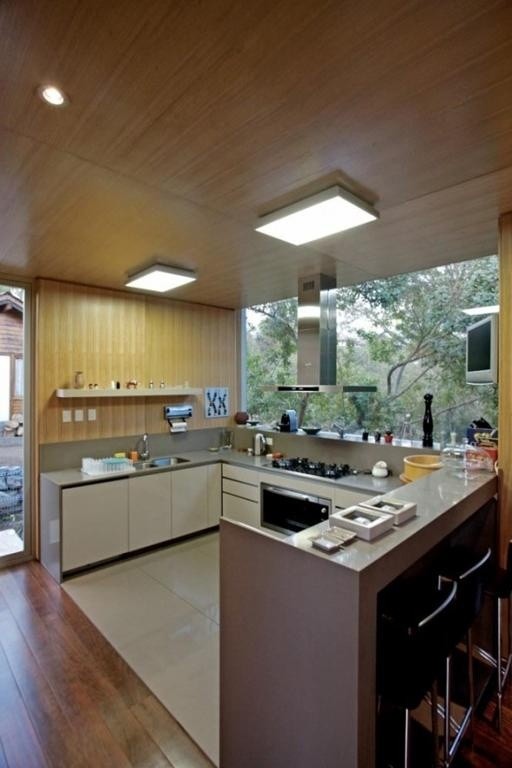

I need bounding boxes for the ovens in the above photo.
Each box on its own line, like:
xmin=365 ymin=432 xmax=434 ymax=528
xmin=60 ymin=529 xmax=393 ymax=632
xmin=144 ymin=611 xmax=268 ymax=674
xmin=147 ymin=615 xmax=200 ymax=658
xmin=260 ymin=484 xmax=331 ymax=535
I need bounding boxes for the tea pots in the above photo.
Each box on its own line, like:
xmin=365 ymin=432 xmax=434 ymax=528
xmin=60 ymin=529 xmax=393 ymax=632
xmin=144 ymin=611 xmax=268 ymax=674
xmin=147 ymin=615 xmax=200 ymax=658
xmin=252 ymin=433 xmax=267 ymax=456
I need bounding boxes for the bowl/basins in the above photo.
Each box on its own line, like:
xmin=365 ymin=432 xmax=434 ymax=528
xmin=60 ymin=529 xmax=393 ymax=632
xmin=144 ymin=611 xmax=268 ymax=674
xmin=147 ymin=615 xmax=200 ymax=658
xmin=403 ymin=455 xmax=441 ymax=481
xmin=246 ymin=421 xmax=260 ymax=425
xmin=302 ymin=427 xmax=322 ymax=434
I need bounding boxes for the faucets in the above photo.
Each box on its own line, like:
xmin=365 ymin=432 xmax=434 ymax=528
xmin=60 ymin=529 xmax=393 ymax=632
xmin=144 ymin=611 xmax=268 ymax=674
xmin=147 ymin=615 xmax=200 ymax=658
xmin=141 ymin=434 xmax=149 ymax=458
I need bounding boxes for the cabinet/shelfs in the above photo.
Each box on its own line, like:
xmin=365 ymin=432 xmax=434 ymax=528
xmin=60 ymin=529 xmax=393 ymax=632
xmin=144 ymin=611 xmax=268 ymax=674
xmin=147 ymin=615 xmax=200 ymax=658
xmin=332 ymin=487 xmax=374 ymax=517
xmin=223 ymin=466 xmax=259 ymax=529
xmin=63 ymin=460 xmax=222 ymax=573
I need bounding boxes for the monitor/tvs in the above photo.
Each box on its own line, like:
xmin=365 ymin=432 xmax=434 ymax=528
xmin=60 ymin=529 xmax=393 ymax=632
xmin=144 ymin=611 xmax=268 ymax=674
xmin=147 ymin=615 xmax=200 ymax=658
xmin=465 ymin=312 xmax=499 ymax=385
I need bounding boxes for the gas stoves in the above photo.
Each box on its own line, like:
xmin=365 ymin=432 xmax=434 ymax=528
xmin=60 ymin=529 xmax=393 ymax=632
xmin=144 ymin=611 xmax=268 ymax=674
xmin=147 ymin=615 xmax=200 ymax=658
xmin=264 ymin=457 xmax=360 ymax=480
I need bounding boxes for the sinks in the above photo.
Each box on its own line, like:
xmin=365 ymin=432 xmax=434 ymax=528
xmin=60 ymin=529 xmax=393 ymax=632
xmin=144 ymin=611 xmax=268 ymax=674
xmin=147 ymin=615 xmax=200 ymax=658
xmin=134 ymin=461 xmax=158 ymax=469
xmin=154 ymin=457 xmax=191 ymax=466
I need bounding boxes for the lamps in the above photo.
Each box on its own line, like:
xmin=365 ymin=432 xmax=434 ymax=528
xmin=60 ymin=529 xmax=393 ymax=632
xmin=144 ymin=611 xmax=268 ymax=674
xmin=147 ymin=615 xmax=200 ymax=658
xmin=126 ymin=266 xmax=197 ymax=295
xmin=251 ymin=186 xmax=382 ymax=246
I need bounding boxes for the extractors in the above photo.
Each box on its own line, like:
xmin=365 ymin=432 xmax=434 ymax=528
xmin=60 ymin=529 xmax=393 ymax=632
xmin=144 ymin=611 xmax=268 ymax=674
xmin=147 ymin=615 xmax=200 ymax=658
xmin=256 ymin=273 xmax=377 ymax=394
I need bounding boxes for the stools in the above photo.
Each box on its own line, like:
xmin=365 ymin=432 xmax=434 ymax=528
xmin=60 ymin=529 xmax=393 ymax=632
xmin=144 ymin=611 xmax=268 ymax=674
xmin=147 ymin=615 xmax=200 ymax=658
xmin=382 ymin=579 xmax=472 ymax=763
xmin=425 ymin=540 xmax=507 ymax=740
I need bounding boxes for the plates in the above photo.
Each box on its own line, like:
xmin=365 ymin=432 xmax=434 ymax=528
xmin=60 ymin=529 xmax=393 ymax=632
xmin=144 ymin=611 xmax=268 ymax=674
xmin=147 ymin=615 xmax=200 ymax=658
xmin=308 ymin=534 xmax=344 ymax=551
xmin=324 ymin=526 xmax=357 ymax=542
